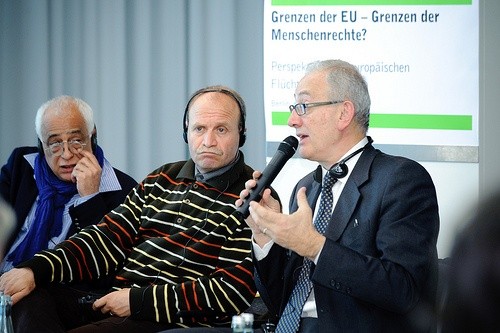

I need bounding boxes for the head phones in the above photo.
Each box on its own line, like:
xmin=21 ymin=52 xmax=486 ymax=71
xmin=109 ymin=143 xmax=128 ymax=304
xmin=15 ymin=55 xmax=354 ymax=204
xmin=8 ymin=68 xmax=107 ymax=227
xmin=183 ymin=88 xmax=246 ymax=147
xmin=316 ymin=140 xmax=373 ymax=183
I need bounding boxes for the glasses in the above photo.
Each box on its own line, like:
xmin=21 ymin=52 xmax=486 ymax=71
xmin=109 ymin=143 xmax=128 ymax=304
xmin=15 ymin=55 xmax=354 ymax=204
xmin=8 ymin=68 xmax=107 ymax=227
xmin=289 ymin=100 xmax=345 ymax=116
xmin=39 ymin=134 xmax=93 ymax=157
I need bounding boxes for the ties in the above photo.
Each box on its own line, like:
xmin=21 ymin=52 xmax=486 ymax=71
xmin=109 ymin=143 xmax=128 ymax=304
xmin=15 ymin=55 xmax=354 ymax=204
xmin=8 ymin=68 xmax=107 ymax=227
xmin=275 ymin=172 xmax=338 ymax=333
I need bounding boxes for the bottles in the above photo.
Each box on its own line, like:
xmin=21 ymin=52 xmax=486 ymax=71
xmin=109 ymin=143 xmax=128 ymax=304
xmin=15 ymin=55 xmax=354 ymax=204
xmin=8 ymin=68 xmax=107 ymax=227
xmin=241 ymin=313 xmax=254 ymax=333
xmin=233 ymin=316 xmax=244 ymax=333
xmin=0 ymin=291 xmax=14 ymax=333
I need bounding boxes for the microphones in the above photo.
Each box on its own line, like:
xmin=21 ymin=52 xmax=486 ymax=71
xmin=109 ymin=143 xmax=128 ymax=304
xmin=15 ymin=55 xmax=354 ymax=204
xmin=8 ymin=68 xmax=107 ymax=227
xmin=236 ymin=135 xmax=298 ymax=218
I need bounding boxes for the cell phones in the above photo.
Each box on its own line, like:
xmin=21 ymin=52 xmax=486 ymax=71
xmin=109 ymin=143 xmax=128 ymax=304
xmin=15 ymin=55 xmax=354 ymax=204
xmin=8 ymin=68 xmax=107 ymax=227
xmin=78 ymin=295 xmax=101 ymax=303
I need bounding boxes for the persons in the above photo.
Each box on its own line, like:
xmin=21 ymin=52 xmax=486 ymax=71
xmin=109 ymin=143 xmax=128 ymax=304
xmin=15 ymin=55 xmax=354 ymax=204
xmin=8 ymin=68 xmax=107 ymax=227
xmin=0 ymin=84 xmax=283 ymax=333
xmin=435 ymin=189 xmax=500 ymax=333
xmin=0 ymin=95 xmax=139 ymax=333
xmin=158 ymin=59 xmax=440 ymax=333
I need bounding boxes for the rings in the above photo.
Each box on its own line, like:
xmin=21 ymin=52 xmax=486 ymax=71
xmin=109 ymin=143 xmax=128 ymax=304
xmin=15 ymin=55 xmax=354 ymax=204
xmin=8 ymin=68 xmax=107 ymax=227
xmin=263 ymin=227 xmax=267 ymax=234
xmin=109 ymin=311 xmax=113 ymax=316
